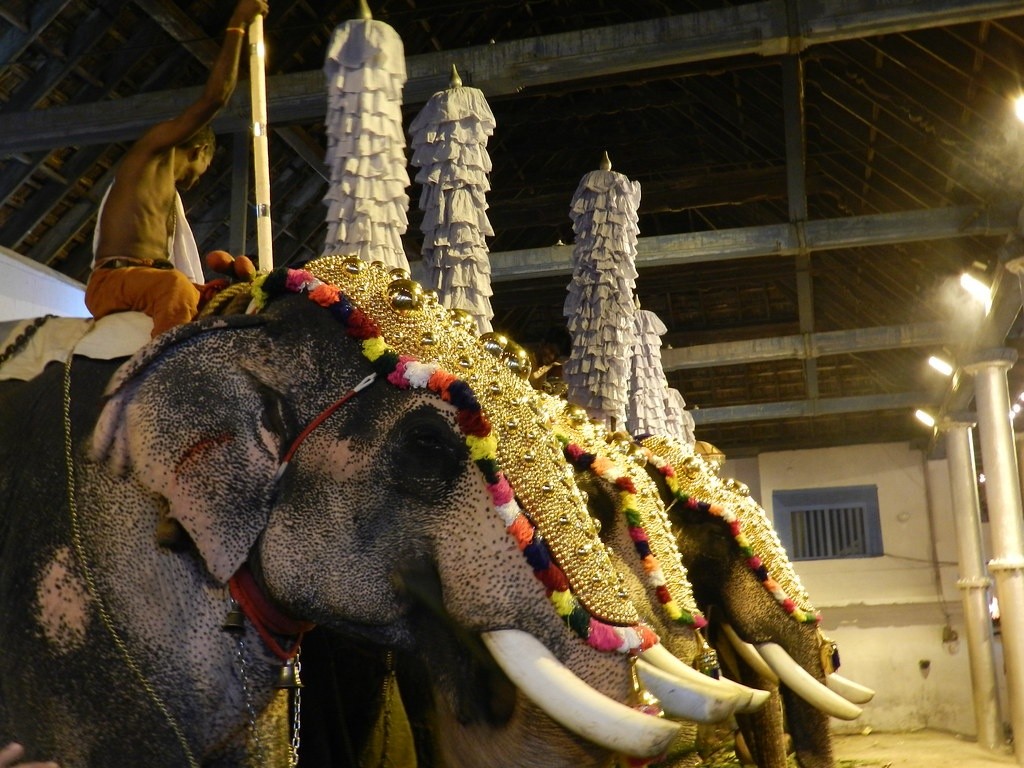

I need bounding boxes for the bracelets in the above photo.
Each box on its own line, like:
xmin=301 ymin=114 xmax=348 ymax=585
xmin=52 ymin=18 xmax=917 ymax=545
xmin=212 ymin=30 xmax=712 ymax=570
xmin=227 ymin=27 xmax=245 ymax=34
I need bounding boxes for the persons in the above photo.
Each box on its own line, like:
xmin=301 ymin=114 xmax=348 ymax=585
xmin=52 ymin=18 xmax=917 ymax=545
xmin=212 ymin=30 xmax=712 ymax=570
xmin=85 ymin=0 xmax=270 ymax=544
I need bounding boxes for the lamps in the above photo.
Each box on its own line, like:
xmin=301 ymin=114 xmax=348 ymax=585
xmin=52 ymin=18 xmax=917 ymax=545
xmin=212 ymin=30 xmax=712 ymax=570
xmin=915 ymin=406 xmax=938 ymax=427
xmin=927 ymin=351 xmax=955 ymax=376
xmin=960 ymin=261 xmax=993 ymax=302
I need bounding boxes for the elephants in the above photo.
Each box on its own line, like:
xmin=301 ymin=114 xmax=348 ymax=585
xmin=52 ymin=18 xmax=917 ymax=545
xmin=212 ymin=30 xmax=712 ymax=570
xmin=1 ymin=252 xmax=875 ymax=768
